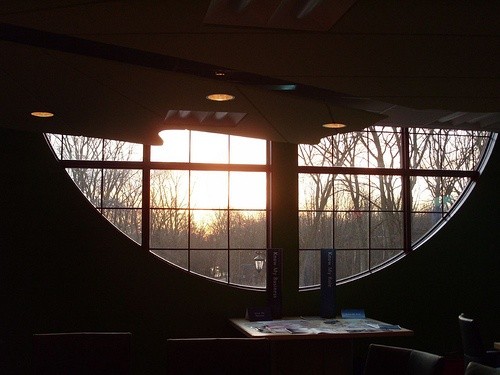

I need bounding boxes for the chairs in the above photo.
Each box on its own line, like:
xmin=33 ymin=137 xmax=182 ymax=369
xmin=167 ymin=337 xmax=271 ymax=375
xmin=362 ymin=344 xmax=445 ymax=375
xmin=32 ymin=332 xmax=129 ymax=375
xmin=456 ymin=313 xmax=500 ymax=375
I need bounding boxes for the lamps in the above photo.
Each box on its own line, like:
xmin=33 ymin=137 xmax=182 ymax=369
xmin=206 ymin=74 xmax=236 ymax=102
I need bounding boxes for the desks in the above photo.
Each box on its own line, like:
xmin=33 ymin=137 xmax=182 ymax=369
xmin=227 ymin=315 xmax=413 ymax=375
